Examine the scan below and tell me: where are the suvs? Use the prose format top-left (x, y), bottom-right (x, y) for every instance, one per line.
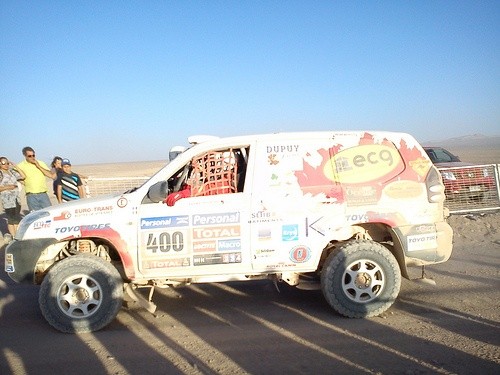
top-left (422, 145), bottom-right (493, 205)
top-left (3, 129), bottom-right (455, 334)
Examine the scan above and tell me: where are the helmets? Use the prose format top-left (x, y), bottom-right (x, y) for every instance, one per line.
top-left (205, 152), bottom-right (235, 178)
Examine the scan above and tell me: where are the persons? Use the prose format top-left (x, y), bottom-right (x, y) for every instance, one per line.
top-left (163, 151), bottom-right (235, 206)
top-left (0, 157), bottom-right (26, 245)
top-left (16, 147), bottom-right (53, 212)
top-left (51, 156), bottom-right (84, 203)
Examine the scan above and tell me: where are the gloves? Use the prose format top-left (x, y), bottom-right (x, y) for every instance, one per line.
top-left (166, 192), bottom-right (185, 206)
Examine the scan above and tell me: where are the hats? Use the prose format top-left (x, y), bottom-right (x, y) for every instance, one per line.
top-left (61, 159), bottom-right (71, 166)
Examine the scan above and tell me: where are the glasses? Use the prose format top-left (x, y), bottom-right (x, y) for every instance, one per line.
top-left (27, 155), bottom-right (35, 158)
top-left (1, 162), bottom-right (9, 165)
